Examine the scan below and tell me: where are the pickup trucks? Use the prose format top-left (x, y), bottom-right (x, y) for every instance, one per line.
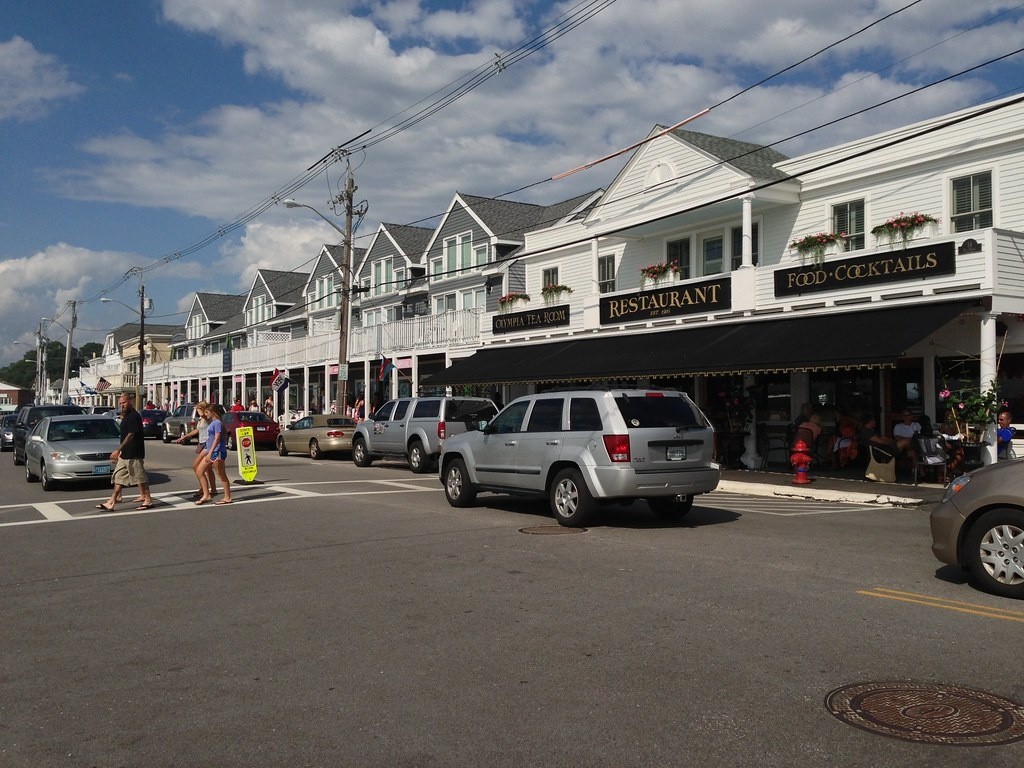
top-left (351, 396), bottom-right (501, 475)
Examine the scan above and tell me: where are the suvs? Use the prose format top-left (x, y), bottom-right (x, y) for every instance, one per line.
top-left (437, 384), bottom-right (721, 528)
top-left (12, 404), bottom-right (89, 467)
top-left (161, 402), bottom-right (200, 445)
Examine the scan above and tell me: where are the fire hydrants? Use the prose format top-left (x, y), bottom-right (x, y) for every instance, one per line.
top-left (788, 438), bottom-right (813, 484)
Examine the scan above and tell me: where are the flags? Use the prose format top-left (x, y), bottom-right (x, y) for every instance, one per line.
top-left (378, 356), bottom-right (395, 381)
top-left (95, 377), bottom-right (111, 391)
top-left (81, 382), bottom-right (97, 395)
top-left (77, 390), bottom-right (84, 402)
top-left (270, 369), bottom-right (290, 393)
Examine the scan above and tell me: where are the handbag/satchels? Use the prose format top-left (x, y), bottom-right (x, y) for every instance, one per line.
top-left (195, 443), bottom-right (205, 454)
top-left (865, 458), bottom-right (896, 483)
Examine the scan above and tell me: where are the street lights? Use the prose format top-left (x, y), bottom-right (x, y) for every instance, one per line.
top-left (99, 297), bottom-right (145, 411)
top-left (13, 340), bottom-right (42, 405)
top-left (282, 197), bottom-right (352, 414)
top-left (41, 317), bottom-right (73, 406)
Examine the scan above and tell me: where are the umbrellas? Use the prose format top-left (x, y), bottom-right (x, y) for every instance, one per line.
top-left (169, 346), bottom-right (177, 361)
top-left (226, 335), bottom-right (233, 350)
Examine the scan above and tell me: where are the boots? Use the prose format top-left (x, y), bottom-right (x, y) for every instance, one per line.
top-left (214, 500), bottom-right (233, 505)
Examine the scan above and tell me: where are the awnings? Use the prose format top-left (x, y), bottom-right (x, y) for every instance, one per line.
top-left (420, 299), bottom-right (980, 389)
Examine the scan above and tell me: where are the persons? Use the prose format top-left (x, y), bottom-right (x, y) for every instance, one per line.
top-left (231, 394), bottom-right (273, 418)
top-left (176, 400), bottom-right (233, 504)
top-left (331, 399), bottom-right (375, 421)
top-left (794, 403), bottom-right (1017, 486)
top-left (95, 392), bottom-right (154, 510)
top-left (146, 394), bottom-right (185, 413)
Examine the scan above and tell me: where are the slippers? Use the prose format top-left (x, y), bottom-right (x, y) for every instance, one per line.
top-left (136, 505), bottom-right (152, 510)
top-left (195, 498), bottom-right (212, 504)
top-left (94, 504), bottom-right (113, 511)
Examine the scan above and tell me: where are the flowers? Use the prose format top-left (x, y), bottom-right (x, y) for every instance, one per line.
top-left (540, 283), bottom-right (575, 308)
top-left (637, 258), bottom-right (683, 295)
top-left (787, 232), bottom-right (846, 272)
top-left (715, 384), bottom-right (753, 432)
top-left (870, 211), bottom-right (942, 253)
top-left (935, 354), bottom-right (1009, 443)
top-left (497, 292), bottom-right (530, 314)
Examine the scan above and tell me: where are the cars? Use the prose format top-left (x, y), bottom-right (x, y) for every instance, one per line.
top-left (275, 414), bottom-right (358, 460)
top-left (81, 406), bottom-right (117, 414)
top-left (138, 409), bottom-right (172, 440)
top-left (929, 454), bottom-right (1024, 599)
top-left (0, 415), bottom-right (17, 452)
top-left (222, 411), bottom-right (280, 451)
top-left (24, 414), bottom-right (124, 491)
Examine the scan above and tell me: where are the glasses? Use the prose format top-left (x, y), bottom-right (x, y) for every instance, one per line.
top-left (998, 419), bottom-right (1004, 421)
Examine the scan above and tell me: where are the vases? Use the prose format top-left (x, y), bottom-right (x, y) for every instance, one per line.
top-left (876, 222), bottom-right (938, 248)
top-left (719, 432), bottom-right (751, 469)
top-left (640, 268), bottom-right (680, 287)
top-left (790, 240), bottom-right (844, 261)
top-left (542, 290), bottom-right (570, 304)
top-left (499, 299), bottom-right (528, 314)
top-left (953, 443), bottom-right (985, 472)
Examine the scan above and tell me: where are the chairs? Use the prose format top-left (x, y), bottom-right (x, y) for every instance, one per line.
top-left (757, 422), bottom-right (789, 470)
top-left (786, 428), bottom-right (818, 474)
top-left (911, 434), bottom-right (949, 486)
